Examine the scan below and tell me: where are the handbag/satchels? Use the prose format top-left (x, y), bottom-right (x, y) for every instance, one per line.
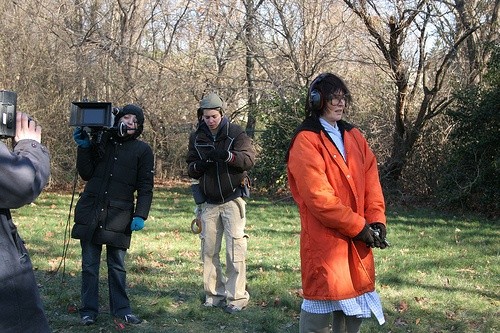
top-left (240, 170), bottom-right (252, 198)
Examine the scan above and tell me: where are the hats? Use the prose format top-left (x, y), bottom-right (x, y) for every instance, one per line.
top-left (199, 93), bottom-right (222, 109)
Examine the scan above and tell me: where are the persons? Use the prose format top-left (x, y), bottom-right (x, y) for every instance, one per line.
top-left (186, 92), bottom-right (255, 314)
top-left (71, 104), bottom-right (154, 325)
top-left (285, 73), bottom-right (387, 333)
top-left (0, 110), bottom-right (54, 333)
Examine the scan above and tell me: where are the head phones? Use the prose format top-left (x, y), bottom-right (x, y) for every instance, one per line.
top-left (196, 96), bottom-right (225, 116)
top-left (307, 72), bottom-right (337, 110)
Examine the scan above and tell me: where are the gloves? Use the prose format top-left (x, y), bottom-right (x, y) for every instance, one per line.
top-left (353, 222), bottom-right (388, 249)
top-left (195, 160), bottom-right (208, 171)
top-left (207, 148), bottom-right (230, 162)
top-left (74, 127), bottom-right (90, 148)
top-left (131, 217), bottom-right (144, 231)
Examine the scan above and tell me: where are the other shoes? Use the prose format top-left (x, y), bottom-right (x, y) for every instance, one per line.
top-left (225, 303), bottom-right (240, 313)
top-left (121, 313), bottom-right (141, 324)
top-left (81, 315), bottom-right (95, 325)
top-left (202, 301), bottom-right (214, 308)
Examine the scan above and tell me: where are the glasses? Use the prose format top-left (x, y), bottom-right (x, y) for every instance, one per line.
top-left (327, 95), bottom-right (347, 106)
